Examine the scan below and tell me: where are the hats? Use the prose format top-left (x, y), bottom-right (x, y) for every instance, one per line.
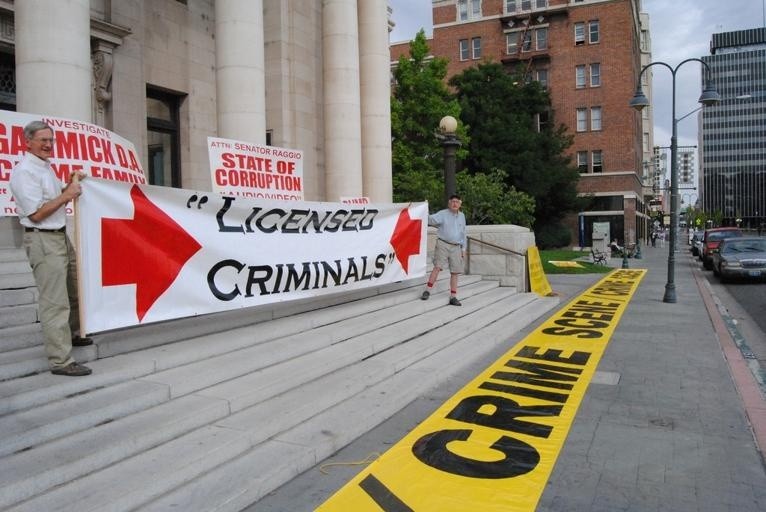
top-left (450, 193), bottom-right (460, 200)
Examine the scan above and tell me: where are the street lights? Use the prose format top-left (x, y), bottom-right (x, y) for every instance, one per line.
top-left (680, 192), bottom-right (701, 220)
top-left (629, 58), bottom-right (722, 305)
top-left (676, 95), bottom-right (752, 132)
top-left (434, 115), bottom-right (461, 211)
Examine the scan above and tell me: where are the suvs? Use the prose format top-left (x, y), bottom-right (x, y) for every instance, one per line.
top-left (700, 227), bottom-right (744, 268)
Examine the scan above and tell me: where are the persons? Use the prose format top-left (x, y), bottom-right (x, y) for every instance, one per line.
top-left (650, 229), bottom-right (657, 248)
top-left (658, 230), bottom-right (666, 248)
top-left (7, 119), bottom-right (95, 377)
top-left (610, 237), bottom-right (625, 254)
top-left (419, 192), bottom-right (469, 307)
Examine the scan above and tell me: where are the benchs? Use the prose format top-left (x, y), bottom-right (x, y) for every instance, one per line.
top-left (591, 248), bottom-right (607, 266)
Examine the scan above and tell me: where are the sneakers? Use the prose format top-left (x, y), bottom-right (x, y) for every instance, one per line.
top-left (420, 291), bottom-right (430, 299)
top-left (71, 336), bottom-right (93, 346)
top-left (52, 361), bottom-right (91, 375)
top-left (450, 297), bottom-right (461, 306)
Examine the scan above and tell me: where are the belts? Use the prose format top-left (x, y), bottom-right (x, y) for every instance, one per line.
top-left (439, 237), bottom-right (459, 246)
top-left (24, 226), bottom-right (66, 233)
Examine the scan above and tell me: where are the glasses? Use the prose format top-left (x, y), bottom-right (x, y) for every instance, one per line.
top-left (33, 137), bottom-right (56, 143)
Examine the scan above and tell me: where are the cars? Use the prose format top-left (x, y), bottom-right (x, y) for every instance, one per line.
top-left (714, 236), bottom-right (766, 284)
top-left (690, 231), bottom-right (704, 261)
